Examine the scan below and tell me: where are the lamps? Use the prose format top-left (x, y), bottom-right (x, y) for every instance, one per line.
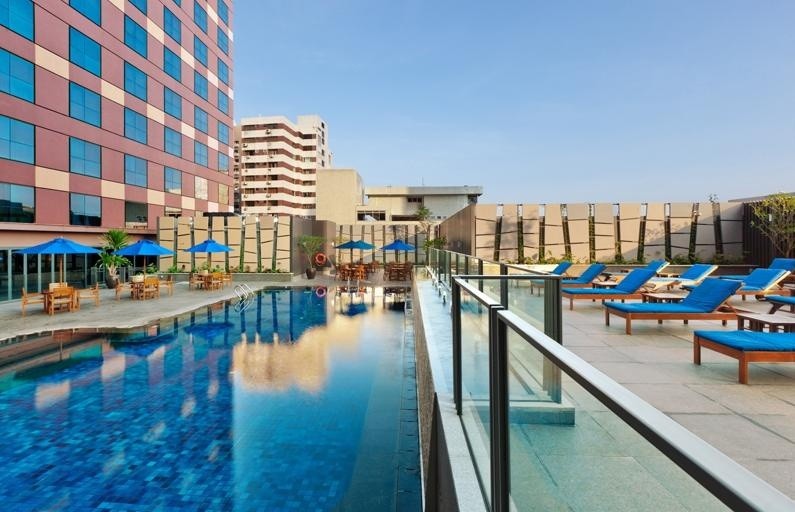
top-left (115, 270), bottom-right (173, 302)
top-left (21, 282), bottom-right (100, 319)
top-left (509, 258), bottom-right (795, 385)
top-left (333, 260), bottom-right (413, 281)
top-left (189, 270), bottom-right (232, 292)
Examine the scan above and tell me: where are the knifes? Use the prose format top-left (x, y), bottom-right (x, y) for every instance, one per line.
top-left (243, 143), bottom-right (248, 147)
top-left (265, 129), bottom-right (270, 133)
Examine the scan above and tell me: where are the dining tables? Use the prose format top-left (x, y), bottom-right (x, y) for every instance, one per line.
top-left (94, 228), bottom-right (134, 288)
top-left (296, 232), bottom-right (329, 279)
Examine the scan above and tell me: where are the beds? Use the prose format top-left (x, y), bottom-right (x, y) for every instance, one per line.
top-left (355, 240), bottom-right (376, 264)
top-left (110, 239), bottom-right (178, 301)
top-left (333, 240), bottom-right (361, 280)
top-left (12, 335), bottom-right (103, 385)
top-left (112, 334), bottom-right (177, 357)
top-left (184, 321), bottom-right (234, 339)
top-left (11, 237), bottom-right (105, 310)
top-left (182, 240), bottom-right (235, 273)
top-left (337, 293), bottom-right (368, 318)
top-left (387, 301), bottom-right (411, 313)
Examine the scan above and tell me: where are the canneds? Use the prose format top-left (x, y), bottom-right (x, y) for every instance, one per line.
top-left (315, 287), bottom-right (327, 298)
top-left (315, 253), bottom-right (326, 264)
